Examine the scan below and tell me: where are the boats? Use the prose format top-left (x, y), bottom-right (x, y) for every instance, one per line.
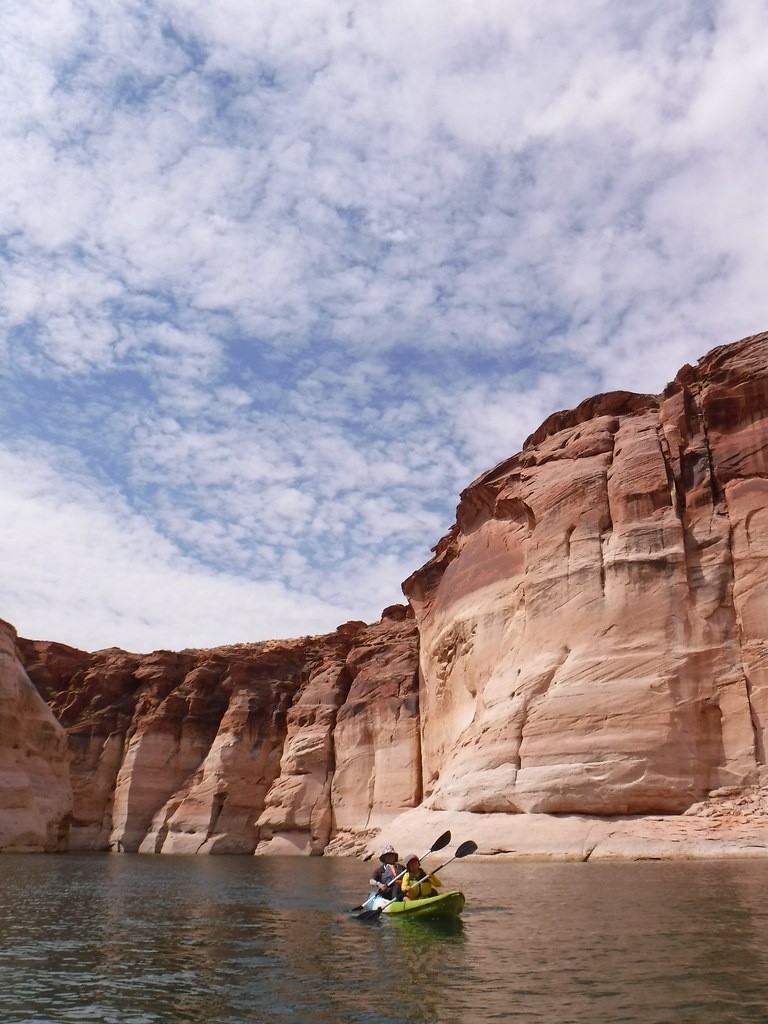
top-left (364, 889), bottom-right (467, 920)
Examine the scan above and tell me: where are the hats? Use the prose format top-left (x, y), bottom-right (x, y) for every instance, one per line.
top-left (379, 844), bottom-right (398, 863)
top-left (405, 854), bottom-right (418, 866)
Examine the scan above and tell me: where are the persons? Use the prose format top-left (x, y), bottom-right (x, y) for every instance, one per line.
top-left (368, 845), bottom-right (441, 902)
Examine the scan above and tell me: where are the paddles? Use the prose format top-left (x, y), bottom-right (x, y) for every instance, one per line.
top-left (351, 830), bottom-right (452, 912)
top-left (357, 840), bottom-right (478, 921)
